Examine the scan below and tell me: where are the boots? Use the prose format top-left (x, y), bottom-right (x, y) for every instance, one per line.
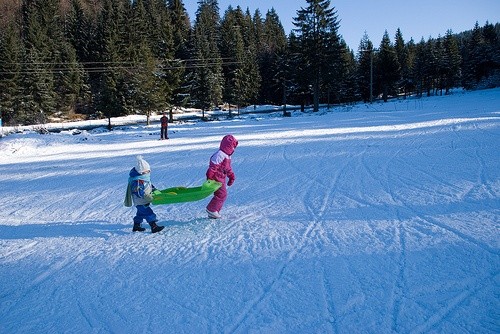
top-left (132, 222), bottom-right (145, 231)
top-left (149, 221), bottom-right (164, 233)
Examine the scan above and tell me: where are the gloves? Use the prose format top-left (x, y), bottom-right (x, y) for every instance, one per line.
top-left (227, 173), bottom-right (235, 186)
top-left (144, 193), bottom-right (154, 202)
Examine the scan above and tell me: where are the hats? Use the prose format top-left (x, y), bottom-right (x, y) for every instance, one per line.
top-left (134, 155), bottom-right (151, 175)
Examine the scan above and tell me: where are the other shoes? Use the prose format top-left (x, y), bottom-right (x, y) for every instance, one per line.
top-left (205, 209), bottom-right (222, 220)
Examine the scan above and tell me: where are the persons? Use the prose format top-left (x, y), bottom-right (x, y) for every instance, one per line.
top-left (206, 134), bottom-right (238, 219)
top-left (128, 155), bottom-right (165, 233)
top-left (160, 113), bottom-right (169, 140)
